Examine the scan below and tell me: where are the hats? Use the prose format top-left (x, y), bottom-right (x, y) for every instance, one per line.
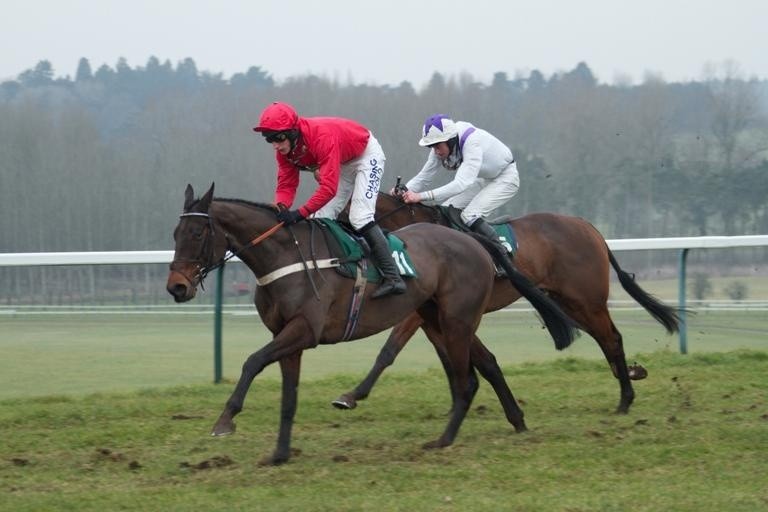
top-left (417, 115), bottom-right (459, 147)
top-left (254, 103), bottom-right (301, 130)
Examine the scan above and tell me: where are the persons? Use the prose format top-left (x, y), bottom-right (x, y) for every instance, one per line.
top-left (253, 103), bottom-right (407, 298)
top-left (391, 115), bottom-right (521, 258)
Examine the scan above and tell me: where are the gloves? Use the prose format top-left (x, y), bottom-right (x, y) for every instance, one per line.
top-left (278, 208), bottom-right (304, 225)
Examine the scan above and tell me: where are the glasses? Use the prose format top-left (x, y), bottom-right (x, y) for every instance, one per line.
top-left (265, 133), bottom-right (287, 143)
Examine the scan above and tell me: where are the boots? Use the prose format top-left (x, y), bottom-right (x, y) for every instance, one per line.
top-left (471, 218), bottom-right (514, 279)
top-left (361, 222), bottom-right (407, 299)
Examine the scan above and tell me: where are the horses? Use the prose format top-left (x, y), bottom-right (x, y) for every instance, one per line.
top-left (165, 181), bottom-right (580, 467)
top-left (331, 187), bottom-right (696, 418)
